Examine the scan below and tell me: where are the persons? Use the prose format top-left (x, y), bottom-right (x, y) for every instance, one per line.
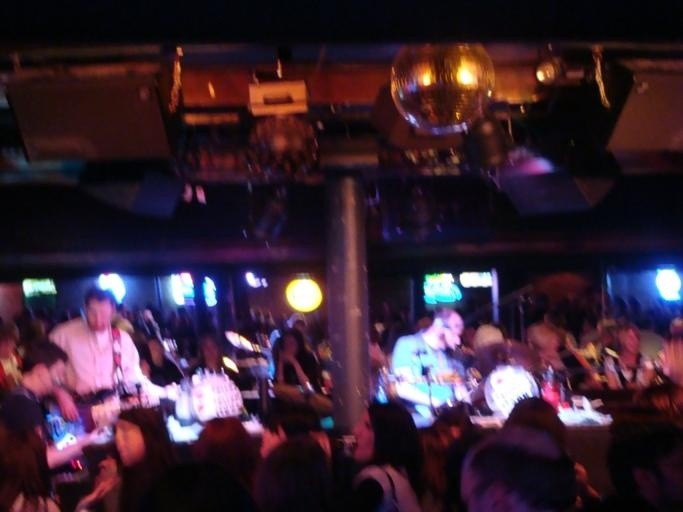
top-left (2, 285), bottom-right (683, 512)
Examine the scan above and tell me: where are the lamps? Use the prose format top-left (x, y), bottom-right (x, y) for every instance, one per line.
top-left (390, 42), bottom-right (495, 135)
top-left (244, 116), bottom-right (318, 181)
top-left (536, 55), bottom-right (569, 85)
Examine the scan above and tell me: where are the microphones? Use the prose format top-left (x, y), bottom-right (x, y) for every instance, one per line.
top-left (142, 307), bottom-right (161, 335)
